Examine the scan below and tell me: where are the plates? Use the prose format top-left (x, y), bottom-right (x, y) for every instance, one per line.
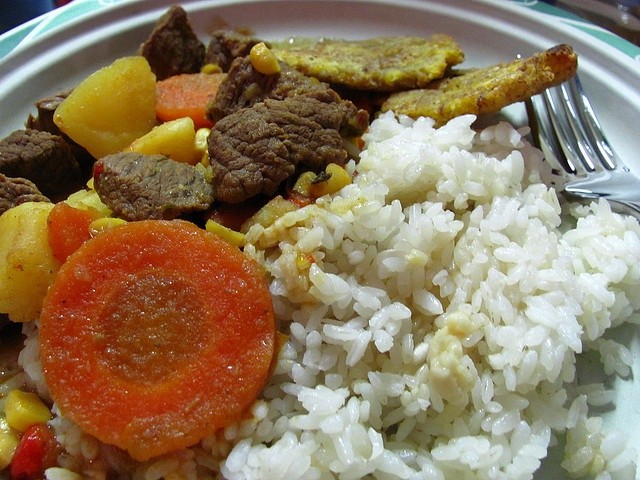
top-left (0, 1), bottom-right (638, 476)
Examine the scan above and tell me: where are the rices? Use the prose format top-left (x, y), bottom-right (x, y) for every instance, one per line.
top-left (19, 109), bottom-right (640, 480)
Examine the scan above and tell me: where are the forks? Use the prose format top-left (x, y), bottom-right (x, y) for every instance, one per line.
top-left (515, 51), bottom-right (640, 223)
